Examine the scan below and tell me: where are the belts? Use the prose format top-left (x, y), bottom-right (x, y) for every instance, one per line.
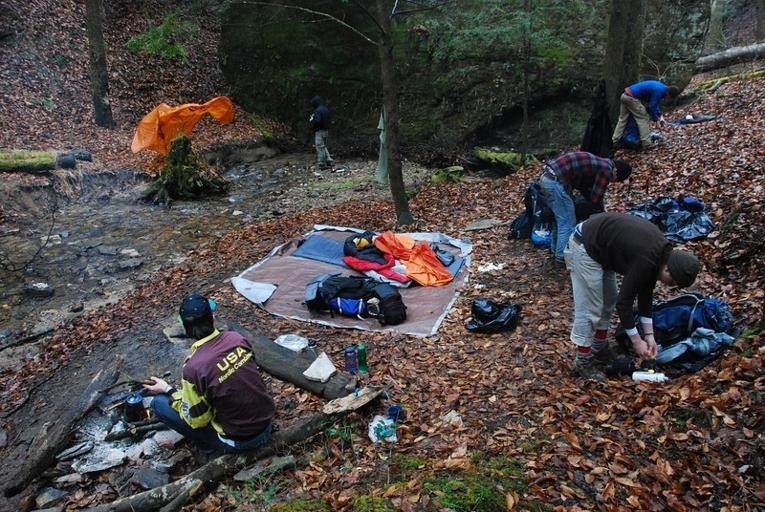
top-left (226, 423), bottom-right (271, 442)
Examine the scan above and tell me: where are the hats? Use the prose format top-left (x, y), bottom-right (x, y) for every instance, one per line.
top-left (614, 160), bottom-right (631, 182)
top-left (669, 86), bottom-right (680, 98)
top-left (178, 294), bottom-right (216, 317)
top-left (667, 250), bottom-right (700, 288)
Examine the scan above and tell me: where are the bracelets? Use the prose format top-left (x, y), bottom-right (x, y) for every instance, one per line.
top-left (643, 332), bottom-right (655, 336)
top-left (167, 388), bottom-right (178, 396)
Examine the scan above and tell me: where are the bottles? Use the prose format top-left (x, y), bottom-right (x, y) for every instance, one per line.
top-left (632, 372), bottom-right (667, 384)
top-left (125, 394), bottom-right (147, 422)
top-left (345, 345), bottom-right (366, 376)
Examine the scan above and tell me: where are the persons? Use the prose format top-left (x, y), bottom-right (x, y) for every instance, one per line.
top-left (611, 79), bottom-right (680, 150)
top-left (306, 95), bottom-right (334, 166)
top-left (540, 151), bottom-right (633, 265)
top-left (141, 292), bottom-right (277, 456)
top-left (561, 209), bottom-right (701, 382)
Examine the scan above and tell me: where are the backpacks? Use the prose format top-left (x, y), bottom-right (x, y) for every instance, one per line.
top-left (616, 292), bottom-right (731, 348)
top-left (303, 272), bottom-right (406, 326)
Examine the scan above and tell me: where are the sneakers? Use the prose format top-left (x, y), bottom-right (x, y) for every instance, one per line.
top-left (551, 254), bottom-right (565, 264)
top-left (572, 339), bottom-right (620, 382)
